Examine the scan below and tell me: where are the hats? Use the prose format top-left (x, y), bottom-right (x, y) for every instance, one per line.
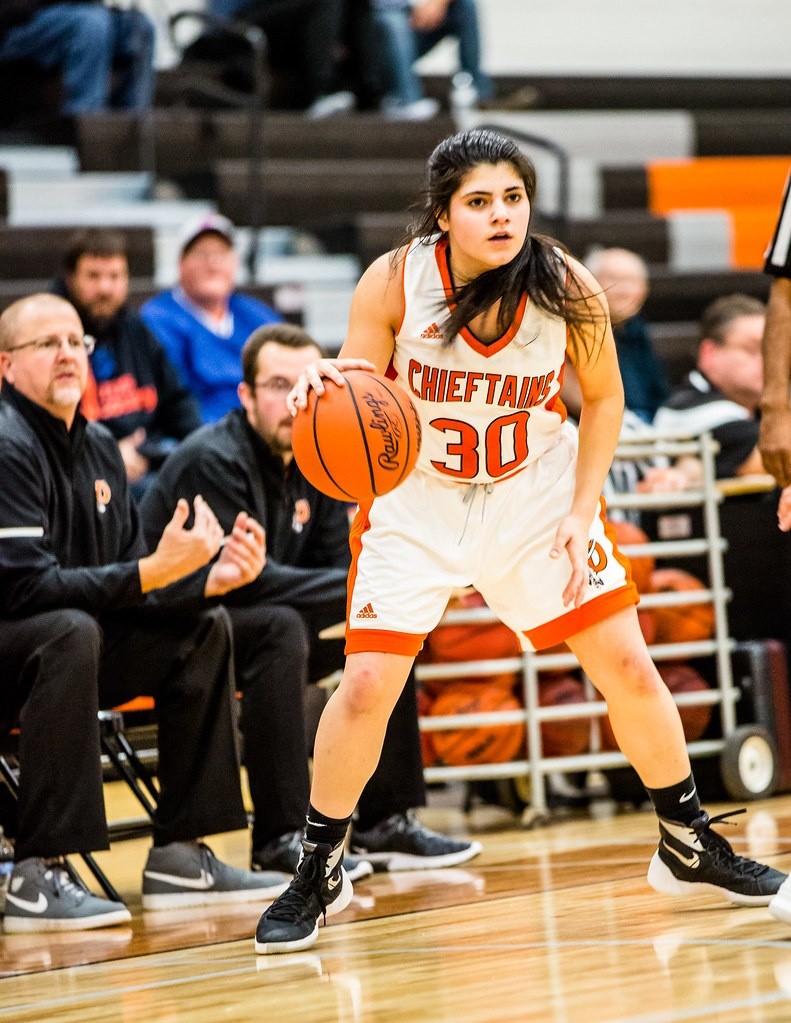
top-left (179, 214), bottom-right (233, 257)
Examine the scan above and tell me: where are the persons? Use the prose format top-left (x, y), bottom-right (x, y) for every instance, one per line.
top-left (0, 213), bottom-right (487, 938)
top-left (0, 0), bottom-right (539, 118)
top-left (252, 129), bottom-right (787, 957)
top-left (559, 168), bottom-right (791, 926)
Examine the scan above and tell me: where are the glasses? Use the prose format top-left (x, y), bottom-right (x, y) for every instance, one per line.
top-left (6, 334), bottom-right (96, 356)
top-left (725, 339), bottom-right (761, 356)
top-left (255, 378), bottom-right (293, 394)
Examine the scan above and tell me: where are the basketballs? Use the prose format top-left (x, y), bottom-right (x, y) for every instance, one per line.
top-left (416, 688), bottom-right (436, 767)
top-left (637, 608), bottom-right (658, 643)
top-left (649, 568), bottom-right (714, 639)
top-left (420, 646), bottom-right (517, 695)
top-left (428, 585), bottom-right (517, 663)
top-left (426, 683), bottom-right (525, 766)
top-left (291, 369), bottom-right (422, 503)
top-left (658, 664), bottom-right (714, 742)
top-left (540, 677), bottom-right (592, 754)
top-left (538, 642), bottom-right (574, 678)
top-left (595, 689), bottom-right (620, 750)
top-left (609, 521), bottom-right (653, 593)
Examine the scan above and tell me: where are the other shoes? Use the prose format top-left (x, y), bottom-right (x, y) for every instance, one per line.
top-left (305, 90), bottom-right (354, 120)
top-left (383, 95), bottom-right (438, 122)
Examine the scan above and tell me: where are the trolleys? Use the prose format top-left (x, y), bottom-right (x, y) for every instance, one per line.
top-left (319, 426), bottom-right (777, 827)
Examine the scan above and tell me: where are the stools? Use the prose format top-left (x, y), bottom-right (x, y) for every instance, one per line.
top-left (1, 709), bottom-right (163, 907)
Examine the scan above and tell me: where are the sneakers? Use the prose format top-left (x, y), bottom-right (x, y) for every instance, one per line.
top-left (647, 808), bottom-right (788, 906)
top-left (344, 807), bottom-right (483, 869)
top-left (768, 872), bottom-right (791, 925)
top-left (255, 834), bottom-right (353, 953)
top-left (249, 828), bottom-right (373, 884)
top-left (4, 858), bottom-right (133, 933)
top-left (141, 844), bottom-right (292, 909)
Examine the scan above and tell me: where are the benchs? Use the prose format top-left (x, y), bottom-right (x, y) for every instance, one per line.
top-left (0, 67), bottom-right (790, 390)
